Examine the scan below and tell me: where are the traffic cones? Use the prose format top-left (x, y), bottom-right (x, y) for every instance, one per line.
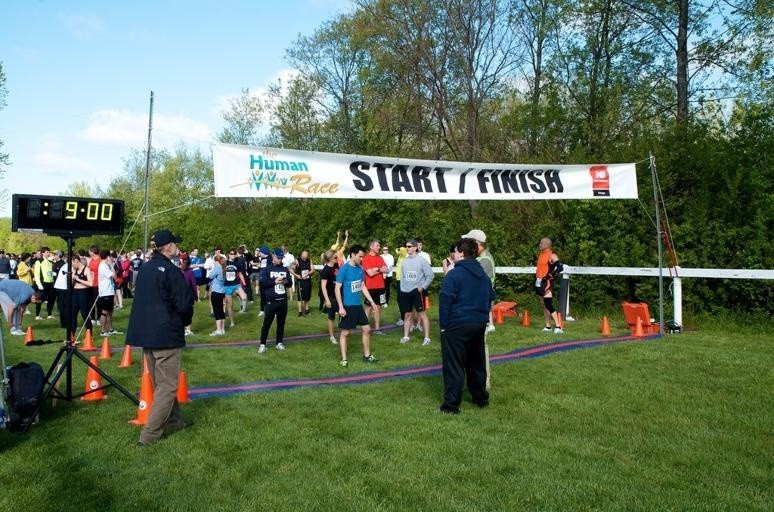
top-left (492, 308), bottom-right (506, 324)
top-left (78, 328), bottom-right (96, 351)
top-left (23, 326), bottom-right (34, 345)
top-left (127, 371), bottom-right (154, 425)
top-left (65, 331), bottom-right (75, 348)
top-left (175, 370), bottom-right (192, 405)
top-left (138, 353), bottom-right (150, 381)
top-left (630, 315), bottom-right (648, 338)
top-left (424, 296), bottom-right (431, 310)
top-left (519, 309), bottom-right (532, 327)
top-left (95, 336), bottom-right (114, 360)
top-left (116, 344), bottom-right (132, 368)
top-left (553, 312), bottom-right (565, 330)
top-left (79, 355), bottom-right (107, 401)
top-left (599, 315), bottom-right (612, 337)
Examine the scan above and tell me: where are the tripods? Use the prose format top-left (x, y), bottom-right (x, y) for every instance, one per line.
top-left (22, 247), bottom-right (140, 433)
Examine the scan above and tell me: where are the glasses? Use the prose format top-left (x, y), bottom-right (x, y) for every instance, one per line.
top-left (405, 246), bottom-right (415, 248)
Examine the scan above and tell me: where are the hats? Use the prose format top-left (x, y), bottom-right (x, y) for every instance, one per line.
top-left (52, 250), bottom-right (63, 257)
top-left (153, 229), bottom-right (183, 247)
top-left (272, 247), bottom-right (285, 258)
top-left (460, 229), bottom-right (486, 243)
top-left (259, 246), bottom-right (270, 255)
top-left (406, 239), bottom-right (419, 252)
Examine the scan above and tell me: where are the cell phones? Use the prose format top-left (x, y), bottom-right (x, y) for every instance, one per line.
top-left (281, 277), bottom-right (286, 281)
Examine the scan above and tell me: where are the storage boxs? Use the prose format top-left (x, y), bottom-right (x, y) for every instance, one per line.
top-left (621, 299), bottom-right (662, 337)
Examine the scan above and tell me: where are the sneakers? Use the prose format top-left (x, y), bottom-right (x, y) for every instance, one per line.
top-left (542, 327), bottom-right (552, 331)
top-left (399, 336), bottom-right (410, 343)
top-left (91, 319), bottom-right (123, 337)
top-left (487, 324), bottom-right (495, 331)
top-left (257, 343), bottom-right (265, 353)
top-left (10, 311), bottom-right (55, 336)
top-left (298, 312), bottom-right (306, 318)
top-left (275, 343), bottom-right (286, 350)
top-left (184, 321), bottom-right (236, 336)
top-left (396, 319), bottom-right (424, 332)
top-left (422, 337), bottom-right (432, 346)
top-left (554, 327), bottom-right (563, 334)
top-left (304, 309), bottom-right (311, 316)
top-left (341, 359), bottom-right (348, 367)
top-left (363, 354), bottom-right (380, 363)
top-left (329, 336), bottom-right (338, 344)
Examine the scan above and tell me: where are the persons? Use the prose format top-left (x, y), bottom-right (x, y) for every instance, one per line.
top-left (438, 238), bottom-right (488, 414)
top-left (319, 230), bottom-right (394, 344)
top-left (443, 244), bottom-right (457, 274)
top-left (287, 251), bottom-right (314, 317)
top-left (172, 244), bottom-right (273, 336)
top-left (334, 245), bottom-right (380, 366)
top-left (395, 240), bottom-right (434, 346)
top-left (258, 247), bottom-right (292, 354)
top-left (0, 245), bottom-right (150, 336)
top-left (534, 238), bottom-right (563, 334)
top-left (461, 229), bottom-right (495, 392)
top-left (125, 230), bottom-right (193, 446)
